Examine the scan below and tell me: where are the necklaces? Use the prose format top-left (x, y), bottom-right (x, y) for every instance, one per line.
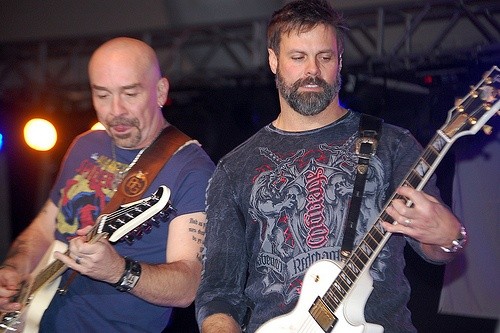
top-left (110, 141), bottom-right (145, 190)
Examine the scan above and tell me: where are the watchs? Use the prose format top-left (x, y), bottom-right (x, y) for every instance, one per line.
top-left (441, 226), bottom-right (468, 255)
top-left (114, 257), bottom-right (141, 292)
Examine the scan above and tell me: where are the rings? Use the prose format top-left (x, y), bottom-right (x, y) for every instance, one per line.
top-left (76, 257), bottom-right (80, 264)
top-left (404, 220), bottom-right (411, 227)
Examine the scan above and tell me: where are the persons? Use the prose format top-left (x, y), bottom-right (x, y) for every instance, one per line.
top-left (195, 0), bottom-right (463, 333)
top-left (0, 37), bottom-right (215, 333)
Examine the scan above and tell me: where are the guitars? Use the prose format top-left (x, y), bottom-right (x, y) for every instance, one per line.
top-left (0, 185), bottom-right (178, 333)
top-left (253, 63), bottom-right (500, 333)
